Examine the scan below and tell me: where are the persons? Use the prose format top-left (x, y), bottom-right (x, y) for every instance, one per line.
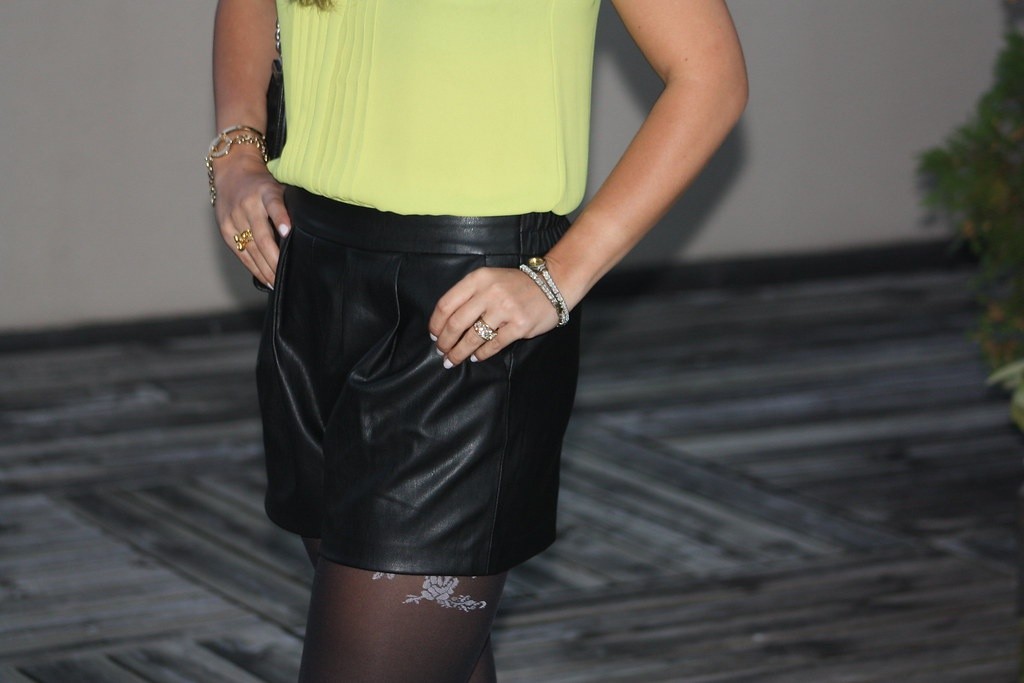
top-left (205, 0), bottom-right (749, 683)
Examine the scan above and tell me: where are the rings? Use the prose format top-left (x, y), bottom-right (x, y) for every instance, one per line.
top-left (234, 229), bottom-right (255, 252)
top-left (473, 315), bottom-right (498, 341)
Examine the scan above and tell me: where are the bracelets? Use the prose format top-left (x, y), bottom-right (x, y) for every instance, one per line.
top-left (519, 257), bottom-right (570, 327)
top-left (206, 125), bottom-right (268, 208)
